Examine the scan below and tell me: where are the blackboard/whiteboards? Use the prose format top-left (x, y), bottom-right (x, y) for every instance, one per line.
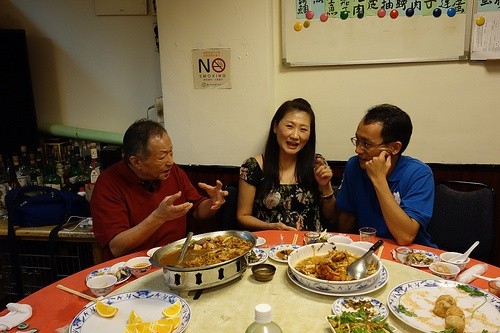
top-left (279, 0), bottom-right (475, 68)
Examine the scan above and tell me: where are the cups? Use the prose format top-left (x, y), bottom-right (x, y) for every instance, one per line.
top-left (395, 246), bottom-right (413, 267)
top-left (304, 232), bottom-right (321, 245)
top-left (487, 279), bottom-right (500, 298)
top-left (359, 227), bottom-right (377, 243)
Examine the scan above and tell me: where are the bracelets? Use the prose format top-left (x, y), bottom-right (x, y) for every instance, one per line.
top-left (320, 190), bottom-right (334, 200)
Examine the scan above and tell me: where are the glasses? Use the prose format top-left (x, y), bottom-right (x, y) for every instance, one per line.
top-left (351, 137), bottom-right (392, 150)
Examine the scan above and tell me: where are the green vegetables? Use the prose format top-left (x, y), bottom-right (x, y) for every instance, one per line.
top-left (431, 328), bottom-right (489, 333)
top-left (398, 305), bottom-right (418, 317)
top-left (456, 283), bottom-right (488, 297)
top-left (327, 309), bottom-right (392, 333)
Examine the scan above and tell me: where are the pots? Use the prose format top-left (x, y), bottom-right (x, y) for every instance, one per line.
top-left (151, 230), bottom-right (257, 290)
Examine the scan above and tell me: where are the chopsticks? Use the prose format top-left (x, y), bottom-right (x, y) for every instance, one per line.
top-left (55, 284), bottom-right (96, 301)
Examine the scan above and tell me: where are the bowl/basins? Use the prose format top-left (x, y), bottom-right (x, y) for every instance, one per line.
top-left (126, 247), bottom-right (162, 277)
top-left (440, 252), bottom-right (470, 271)
top-left (327, 236), bottom-right (374, 251)
top-left (325, 315), bottom-right (397, 333)
top-left (86, 275), bottom-right (117, 297)
top-left (252, 263), bottom-right (276, 281)
top-left (287, 242), bottom-right (382, 292)
top-left (429, 262), bottom-right (461, 281)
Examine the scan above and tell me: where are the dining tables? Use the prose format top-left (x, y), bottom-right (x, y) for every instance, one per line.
top-left (0, 229), bottom-right (500, 333)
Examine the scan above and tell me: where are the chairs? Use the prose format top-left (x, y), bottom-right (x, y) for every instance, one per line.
top-left (427, 180), bottom-right (494, 265)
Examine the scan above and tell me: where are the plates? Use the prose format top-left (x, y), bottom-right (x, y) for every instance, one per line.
top-left (286, 266), bottom-right (389, 297)
top-left (331, 296), bottom-right (389, 322)
top-left (247, 237), bottom-right (303, 264)
top-left (69, 289), bottom-right (191, 333)
top-left (86, 266), bottom-right (131, 285)
top-left (392, 249), bottom-right (439, 268)
top-left (387, 279), bottom-right (500, 333)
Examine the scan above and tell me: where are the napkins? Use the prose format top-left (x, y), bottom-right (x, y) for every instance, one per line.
top-left (0, 303), bottom-right (32, 331)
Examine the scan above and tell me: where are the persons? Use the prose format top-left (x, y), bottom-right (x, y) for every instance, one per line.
top-left (90, 118), bottom-right (229, 261)
top-left (237, 97), bottom-right (337, 231)
top-left (334, 103), bottom-right (438, 248)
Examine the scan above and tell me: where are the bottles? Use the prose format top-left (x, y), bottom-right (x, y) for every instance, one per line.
top-left (245, 304), bottom-right (282, 333)
top-left (0, 140), bottom-right (102, 221)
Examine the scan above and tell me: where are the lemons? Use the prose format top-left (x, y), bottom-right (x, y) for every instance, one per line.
top-left (96, 301), bottom-right (119, 317)
top-left (125, 300), bottom-right (185, 333)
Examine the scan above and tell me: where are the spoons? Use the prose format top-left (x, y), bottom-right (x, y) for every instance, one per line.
top-left (447, 241), bottom-right (479, 263)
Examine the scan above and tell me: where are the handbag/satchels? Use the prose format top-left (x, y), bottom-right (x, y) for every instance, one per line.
top-left (5, 185), bottom-right (87, 228)
